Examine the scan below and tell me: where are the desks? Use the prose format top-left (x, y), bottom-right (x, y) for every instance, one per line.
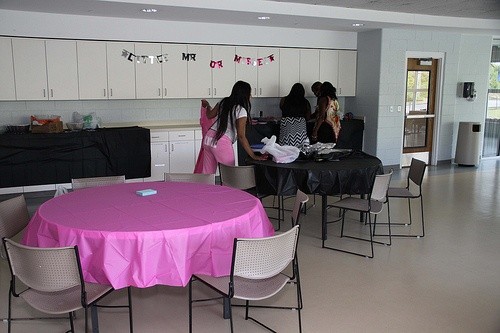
top-left (246, 150), bottom-right (383, 241)
top-left (22, 182), bottom-right (275, 291)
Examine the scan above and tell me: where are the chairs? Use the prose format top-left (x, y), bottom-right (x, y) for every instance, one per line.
top-left (0, 158), bottom-right (428, 333)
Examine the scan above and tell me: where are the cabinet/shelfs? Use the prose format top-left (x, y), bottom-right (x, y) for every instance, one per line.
top-left (97, 120), bottom-right (240, 181)
top-left (0, 34), bottom-right (357, 102)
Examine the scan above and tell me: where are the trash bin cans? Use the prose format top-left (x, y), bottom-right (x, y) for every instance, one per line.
top-left (454, 121), bottom-right (482, 166)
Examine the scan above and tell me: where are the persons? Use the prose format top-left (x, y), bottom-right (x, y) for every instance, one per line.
top-left (309, 82), bottom-right (342, 145)
top-left (279, 83), bottom-right (311, 148)
top-left (201, 81), bottom-right (268, 174)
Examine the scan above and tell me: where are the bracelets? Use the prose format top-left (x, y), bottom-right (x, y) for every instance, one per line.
top-left (205, 105), bottom-right (210, 108)
